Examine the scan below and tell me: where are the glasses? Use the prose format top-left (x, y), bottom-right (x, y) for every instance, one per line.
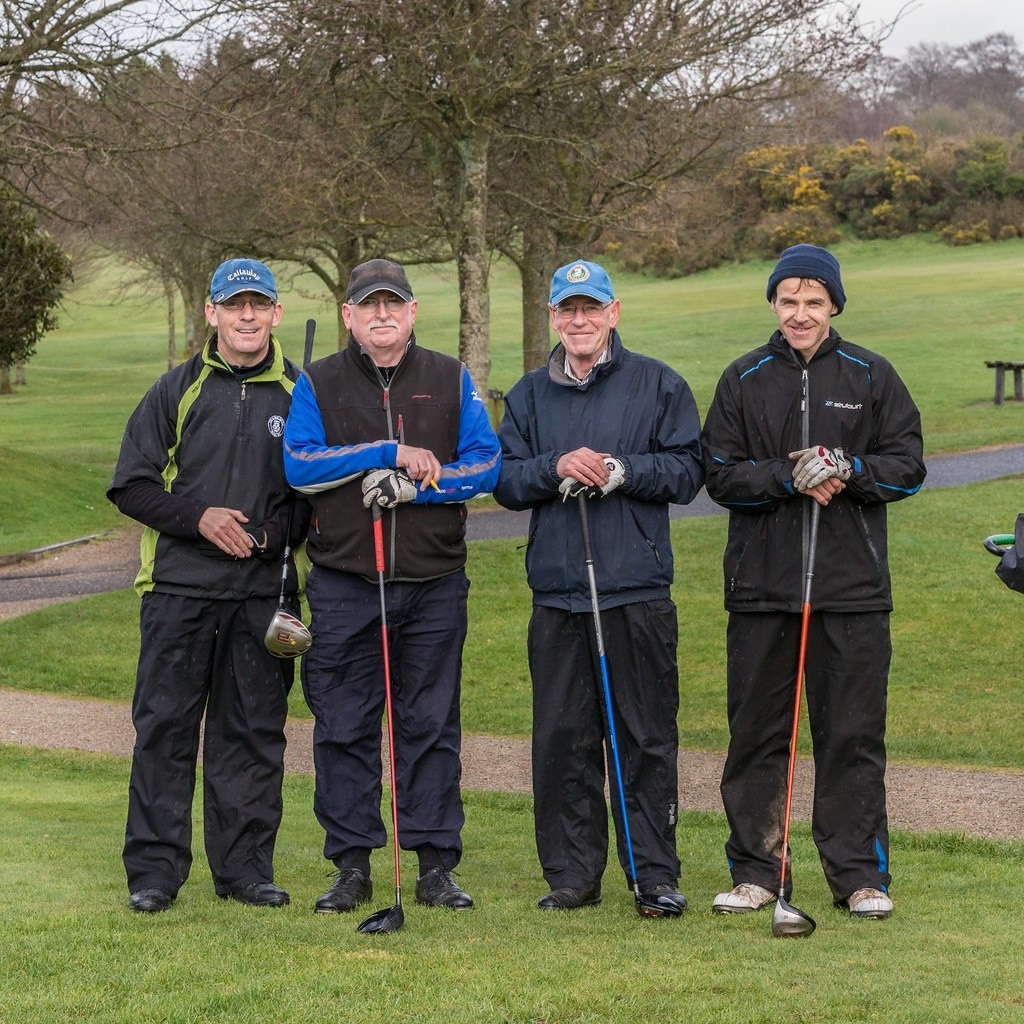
top-left (348, 297), bottom-right (405, 312)
top-left (552, 301), bottom-right (614, 319)
top-left (215, 297), bottom-right (275, 311)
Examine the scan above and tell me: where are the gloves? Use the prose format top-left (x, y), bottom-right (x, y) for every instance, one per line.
top-left (558, 458), bottom-right (626, 504)
top-left (361, 468), bottom-right (417, 509)
top-left (789, 445), bottom-right (852, 491)
top-left (198, 524), bottom-right (268, 562)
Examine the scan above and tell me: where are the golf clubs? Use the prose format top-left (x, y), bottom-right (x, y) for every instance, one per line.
top-left (354, 494), bottom-right (405, 937)
top-left (263, 318), bottom-right (316, 660)
top-left (770, 498), bottom-right (822, 940)
top-left (574, 492), bottom-right (690, 918)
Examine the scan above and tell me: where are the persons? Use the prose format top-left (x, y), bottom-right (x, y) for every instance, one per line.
top-left (283, 259), bottom-right (501, 917)
top-left (699, 243), bottom-right (927, 919)
top-left (494, 261), bottom-right (706, 915)
top-left (105, 258), bottom-right (312, 913)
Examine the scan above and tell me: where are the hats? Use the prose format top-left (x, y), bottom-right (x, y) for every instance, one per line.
top-left (346, 259), bottom-right (414, 305)
top-left (549, 259), bottom-right (615, 307)
top-left (767, 244), bottom-right (847, 317)
top-left (210, 258), bottom-right (277, 304)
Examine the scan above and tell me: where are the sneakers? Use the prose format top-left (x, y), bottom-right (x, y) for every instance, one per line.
top-left (644, 884), bottom-right (687, 909)
top-left (414, 866), bottom-right (473, 907)
top-left (220, 881), bottom-right (290, 906)
top-left (316, 868), bottom-right (374, 914)
top-left (847, 888), bottom-right (893, 919)
top-left (712, 883), bottom-right (777, 913)
top-left (129, 888), bottom-right (174, 911)
top-left (538, 888), bottom-right (602, 909)
